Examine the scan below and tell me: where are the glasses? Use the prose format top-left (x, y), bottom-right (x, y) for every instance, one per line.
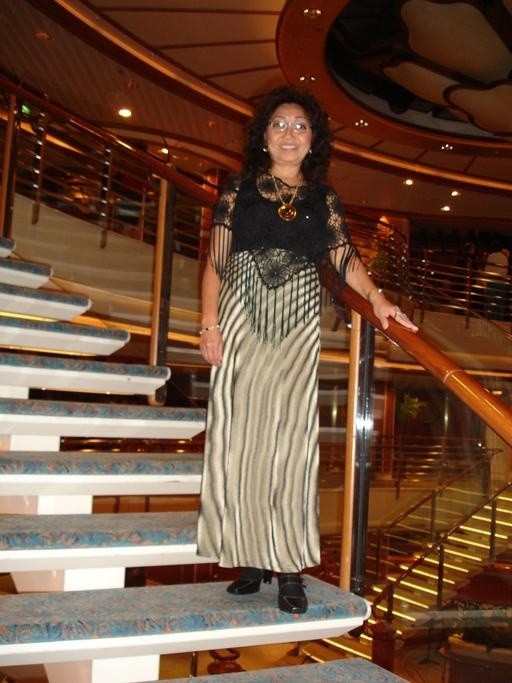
top-left (265, 117), bottom-right (313, 133)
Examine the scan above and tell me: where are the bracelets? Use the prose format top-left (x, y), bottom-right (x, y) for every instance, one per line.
top-left (367, 286), bottom-right (384, 305)
top-left (198, 324), bottom-right (220, 335)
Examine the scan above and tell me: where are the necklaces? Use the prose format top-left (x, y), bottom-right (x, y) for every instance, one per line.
top-left (266, 160), bottom-right (307, 222)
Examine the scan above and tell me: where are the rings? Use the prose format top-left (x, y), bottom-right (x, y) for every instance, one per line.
top-left (393, 310), bottom-right (401, 319)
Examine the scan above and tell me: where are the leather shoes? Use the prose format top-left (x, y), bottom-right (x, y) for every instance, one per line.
top-left (277, 572), bottom-right (308, 614)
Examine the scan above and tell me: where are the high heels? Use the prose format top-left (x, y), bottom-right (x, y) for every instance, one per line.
top-left (226, 564), bottom-right (273, 595)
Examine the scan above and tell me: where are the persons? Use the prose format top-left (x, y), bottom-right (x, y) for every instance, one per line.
top-left (196, 81), bottom-right (421, 616)
top-left (484, 247), bottom-right (510, 319)
top-left (441, 240), bottom-right (482, 317)
